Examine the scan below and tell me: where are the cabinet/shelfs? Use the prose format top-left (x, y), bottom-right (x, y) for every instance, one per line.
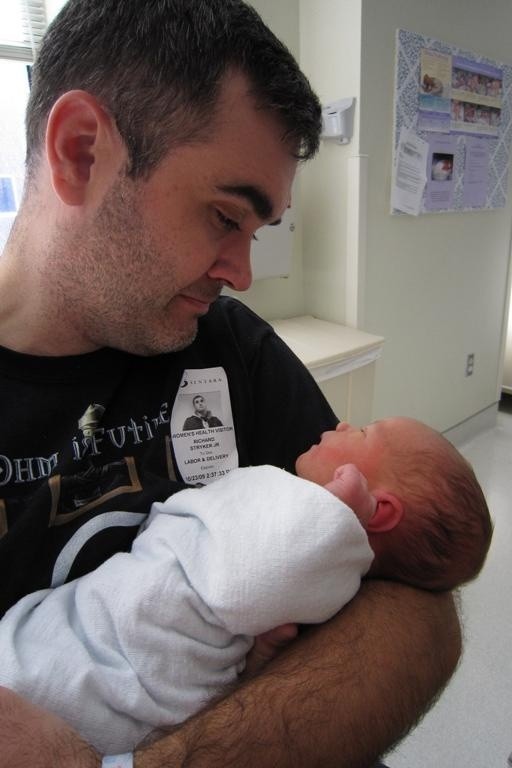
top-left (268, 315), bottom-right (387, 436)
